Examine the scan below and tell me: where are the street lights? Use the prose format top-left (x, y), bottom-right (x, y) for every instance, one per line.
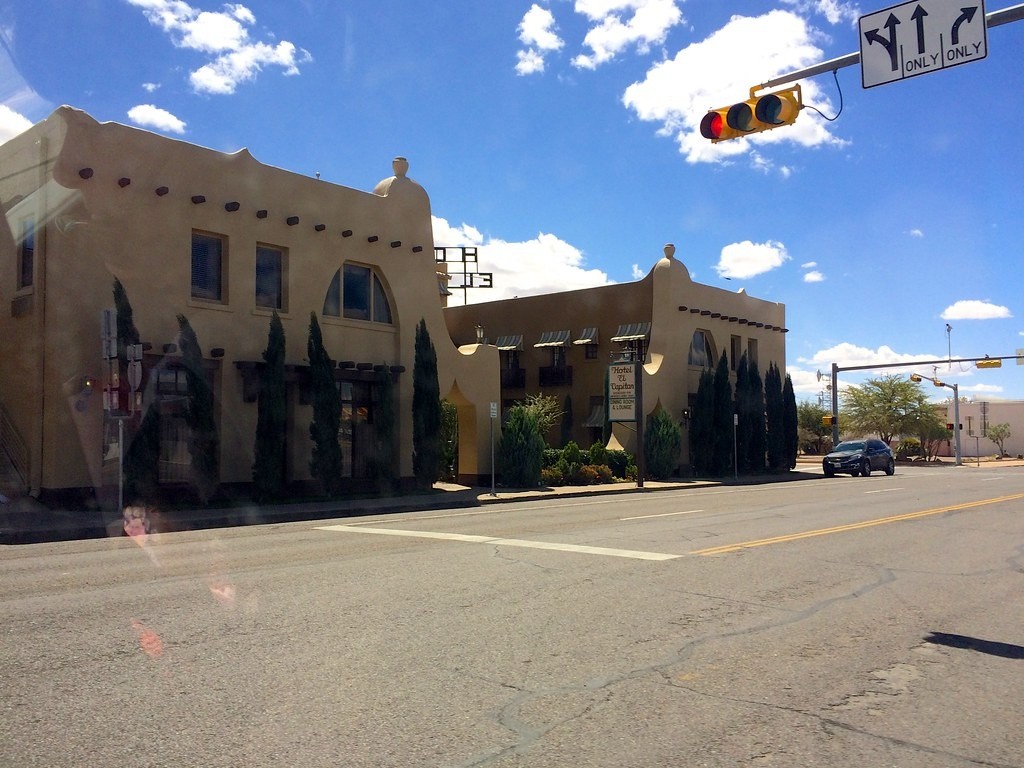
top-left (475, 324), bottom-right (486, 344)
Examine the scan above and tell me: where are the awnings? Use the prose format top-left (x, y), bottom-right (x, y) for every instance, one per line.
top-left (495, 334), bottom-right (525, 351)
top-left (534, 331), bottom-right (573, 348)
top-left (610, 321), bottom-right (652, 344)
top-left (573, 327), bottom-right (600, 345)
top-left (581, 404), bottom-right (606, 428)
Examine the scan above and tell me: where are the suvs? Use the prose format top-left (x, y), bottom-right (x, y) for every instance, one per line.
top-left (823, 438), bottom-right (895, 477)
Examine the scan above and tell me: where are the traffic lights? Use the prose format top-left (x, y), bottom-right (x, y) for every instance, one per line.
top-left (700, 83), bottom-right (804, 143)
top-left (947, 424), bottom-right (954, 430)
